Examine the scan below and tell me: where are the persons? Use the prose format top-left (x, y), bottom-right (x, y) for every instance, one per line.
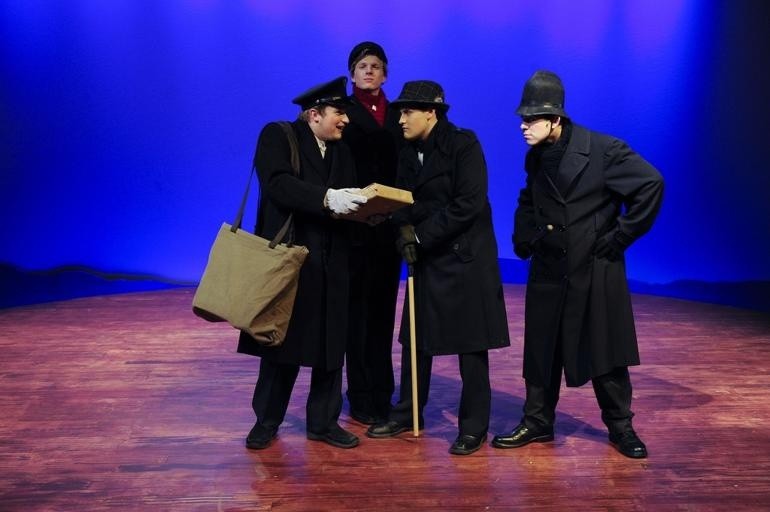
top-left (491, 70), bottom-right (665, 458)
top-left (236, 75), bottom-right (395, 450)
top-left (330, 42), bottom-right (405, 426)
top-left (364, 80), bottom-right (512, 456)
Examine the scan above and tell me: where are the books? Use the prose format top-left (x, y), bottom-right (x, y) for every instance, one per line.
top-left (332, 183), bottom-right (414, 227)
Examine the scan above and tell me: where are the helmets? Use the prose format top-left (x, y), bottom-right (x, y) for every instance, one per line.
top-left (517, 70), bottom-right (571, 118)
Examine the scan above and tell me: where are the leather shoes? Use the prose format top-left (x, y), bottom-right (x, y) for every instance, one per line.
top-left (245, 421), bottom-right (279, 449)
top-left (448, 431), bottom-right (487, 455)
top-left (306, 423), bottom-right (359, 449)
top-left (367, 410), bottom-right (425, 438)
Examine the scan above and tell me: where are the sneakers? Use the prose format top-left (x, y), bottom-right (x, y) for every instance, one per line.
top-left (491, 423), bottom-right (554, 449)
top-left (608, 426), bottom-right (648, 458)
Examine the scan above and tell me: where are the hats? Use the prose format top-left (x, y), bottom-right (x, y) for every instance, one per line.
top-left (388, 80), bottom-right (450, 111)
top-left (348, 41), bottom-right (387, 72)
top-left (291, 75), bottom-right (356, 112)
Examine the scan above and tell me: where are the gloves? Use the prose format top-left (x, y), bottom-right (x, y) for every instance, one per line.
top-left (395, 224), bottom-right (416, 251)
top-left (324, 187), bottom-right (367, 216)
top-left (592, 226), bottom-right (634, 259)
top-left (402, 244), bottom-right (418, 265)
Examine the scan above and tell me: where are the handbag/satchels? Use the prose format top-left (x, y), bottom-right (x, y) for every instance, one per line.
top-left (191, 221), bottom-right (309, 348)
